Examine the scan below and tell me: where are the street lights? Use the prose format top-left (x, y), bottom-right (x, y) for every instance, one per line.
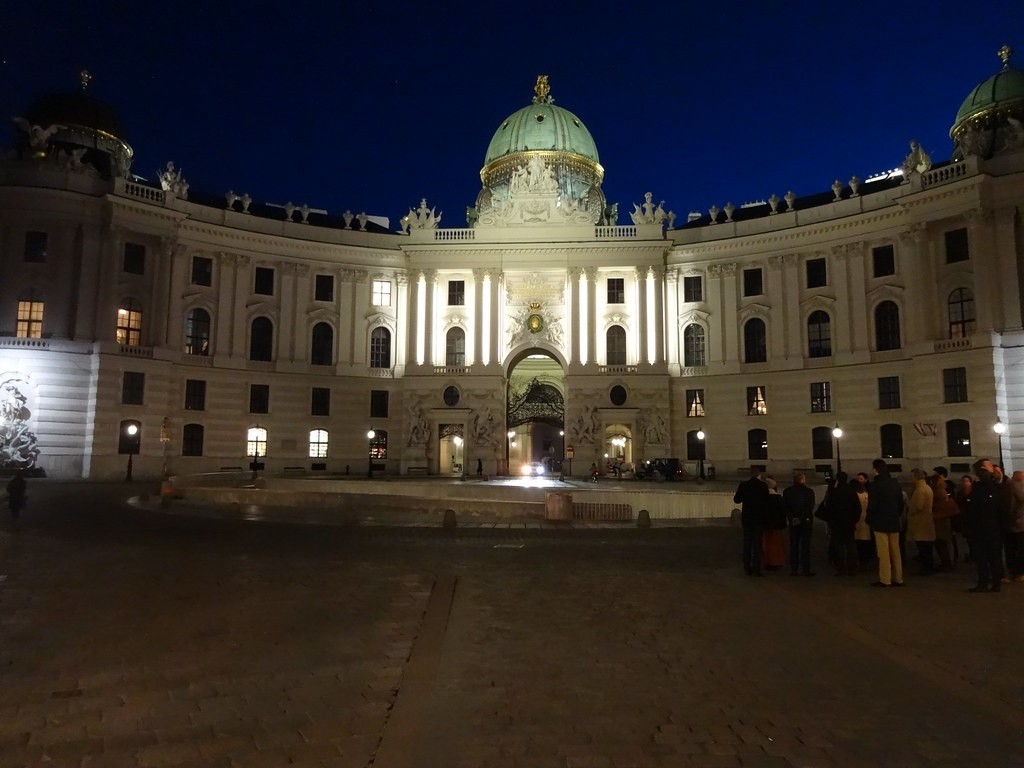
top-left (126, 424), bottom-right (138, 483)
top-left (995, 416), bottom-right (1006, 477)
top-left (696, 427), bottom-right (707, 482)
top-left (831, 422), bottom-right (843, 475)
top-left (367, 425), bottom-right (375, 480)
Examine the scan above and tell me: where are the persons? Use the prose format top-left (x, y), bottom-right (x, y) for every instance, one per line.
top-left (511, 153), bottom-right (558, 192)
top-left (906, 140), bottom-right (923, 174)
top-left (587, 458), bottom-right (668, 483)
top-left (732, 460), bottom-right (1024, 593)
top-left (164, 162), bottom-right (189, 201)
top-left (7, 470), bottom-right (26, 517)
top-left (642, 192), bottom-right (655, 223)
top-left (477, 458), bottom-right (482, 478)
top-left (417, 199), bottom-right (431, 225)
top-left (450, 455), bottom-right (455, 472)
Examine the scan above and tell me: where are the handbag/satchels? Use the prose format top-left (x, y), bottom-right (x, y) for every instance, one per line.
top-left (161, 487), bottom-right (173, 497)
top-left (933, 487), bottom-right (960, 519)
top-left (814, 488), bottom-right (841, 522)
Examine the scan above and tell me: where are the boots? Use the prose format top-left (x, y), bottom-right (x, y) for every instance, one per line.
top-left (968, 570), bottom-right (987, 592)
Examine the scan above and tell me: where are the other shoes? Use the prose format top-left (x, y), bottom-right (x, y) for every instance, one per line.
top-left (935, 564), bottom-right (950, 573)
top-left (801, 572), bottom-right (815, 576)
top-left (920, 569), bottom-right (934, 576)
top-left (790, 572), bottom-right (798, 576)
top-left (893, 581), bottom-right (903, 586)
top-left (748, 569), bottom-right (753, 575)
top-left (880, 581), bottom-right (891, 589)
top-left (754, 572), bottom-right (764, 576)
top-left (1002, 572), bottom-right (1024, 583)
